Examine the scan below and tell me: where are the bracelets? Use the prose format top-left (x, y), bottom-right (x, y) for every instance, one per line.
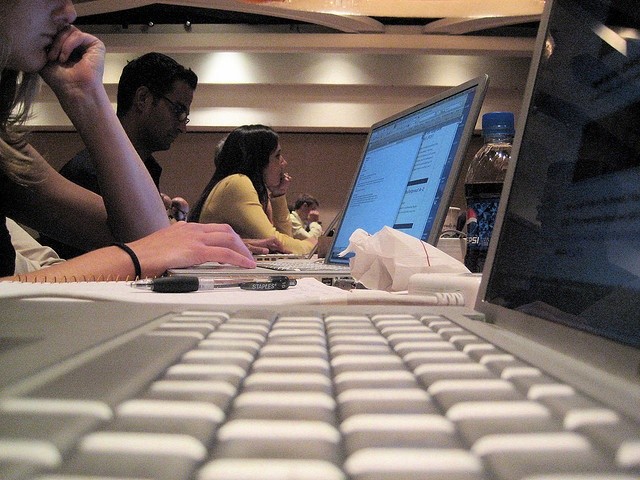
top-left (110, 240), bottom-right (142, 280)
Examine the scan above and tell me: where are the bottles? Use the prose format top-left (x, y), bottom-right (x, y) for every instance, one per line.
top-left (463, 111), bottom-right (515, 273)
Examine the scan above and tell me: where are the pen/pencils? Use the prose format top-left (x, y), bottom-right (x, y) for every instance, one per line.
top-left (131, 276), bottom-right (298, 291)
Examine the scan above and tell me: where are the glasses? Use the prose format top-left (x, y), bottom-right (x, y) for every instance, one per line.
top-left (176, 207), bottom-right (188, 216)
top-left (151, 87), bottom-right (190, 124)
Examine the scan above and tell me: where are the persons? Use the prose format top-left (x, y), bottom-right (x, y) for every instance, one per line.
top-left (188, 123), bottom-right (318, 257)
top-left (1, 0), bottom-right (257, 282)
top-left (511, 158), bottom-right (620, 310)
top-left (157, 191), bottom-right (191, 224)
top-left (215, 133), bottom-right (273, 227)
top-left (287, 196), bottom-right (324, 241)
top-left (37, 50), bottom-right (292, 264)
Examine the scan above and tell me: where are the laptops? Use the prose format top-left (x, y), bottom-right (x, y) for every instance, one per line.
top-left (2, 1), bottom-right (639, 478)
top-left (252, 206), bottom-right (344, 259)
top-left (166, 73), bottom-right (489, 278)
